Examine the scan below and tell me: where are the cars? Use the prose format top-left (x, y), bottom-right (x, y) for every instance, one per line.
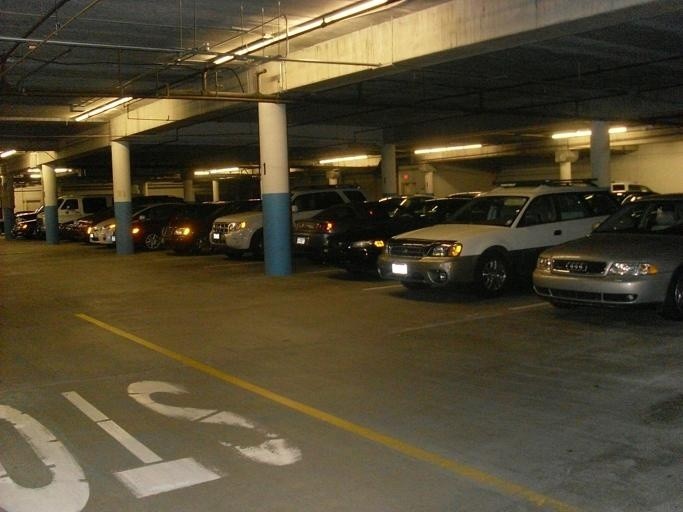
top-left (0, 194), bottom-right (262, 255)
top-left (532, 192), bottom-right (682, 323)
top-left (292, 196), bottom-right (473, 276)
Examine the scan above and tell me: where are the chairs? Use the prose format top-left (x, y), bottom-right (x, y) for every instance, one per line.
top-left (495, 201), bottom-right (598, 227)
top-left (651, 206), bottom-right (680, 231)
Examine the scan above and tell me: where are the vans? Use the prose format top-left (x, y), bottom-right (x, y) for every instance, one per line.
top-left (610, 183), bottom-right (654, 196)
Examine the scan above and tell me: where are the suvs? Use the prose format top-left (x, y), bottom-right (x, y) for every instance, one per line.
top-left (208, 185), bottom-right (368, 262)
top-left (375, 178), bottom-right (622, 300)
